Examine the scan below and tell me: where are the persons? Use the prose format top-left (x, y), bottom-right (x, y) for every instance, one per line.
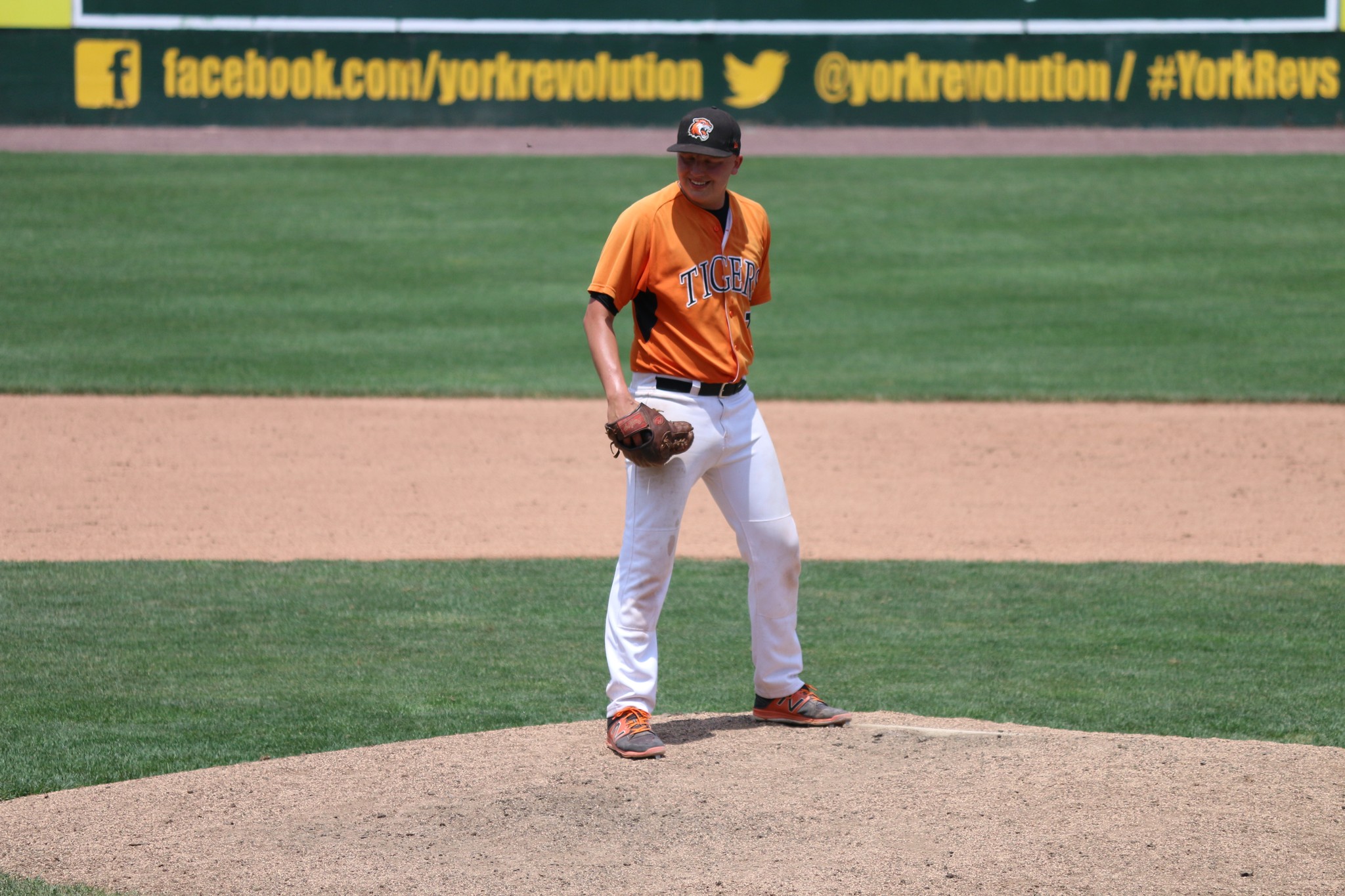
top-left (582, 110), bottom-right (852, 760)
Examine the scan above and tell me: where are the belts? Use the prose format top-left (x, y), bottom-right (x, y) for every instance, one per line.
top-left (655, 377), bottom-right (746, 398)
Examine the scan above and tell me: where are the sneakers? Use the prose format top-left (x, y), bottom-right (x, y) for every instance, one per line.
top-left (606, 706), bottom-right (666, 758)
top-left (752, 684), bottom-right (852, 725)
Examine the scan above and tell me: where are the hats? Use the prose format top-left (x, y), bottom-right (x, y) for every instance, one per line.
top-left (667, 106), bottom-right (741, 157)
